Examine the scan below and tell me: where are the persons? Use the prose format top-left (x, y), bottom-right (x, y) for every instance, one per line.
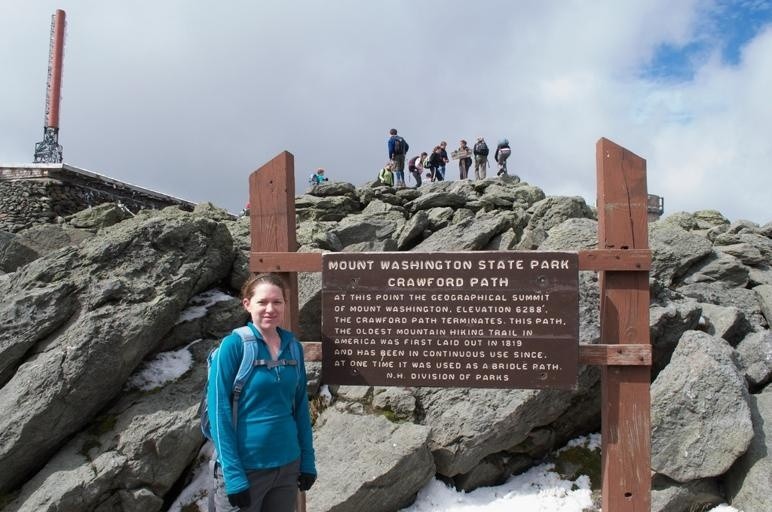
top-left (495, 138), bottom-right (511, 176)
top-left (438, 141), bottom-right (449, 180)
top-left (411, 152), bottom-right (426, 186)
top-left (430, 146), bottom-right (445, 183)
top-left (387, 128), bottom-right (408, 187)
top-left (422, 173), bottom-right (432, 182)
top-left (458, 139), bottom-right (473, 179)
top-left (377, 163), bottom-right (395, 187)
top-left (207, 273), bottom-right (317, 512)
top-left (316, 168), bottom-right (328, 183)
top-left (474, 136), bottom-right (490, 181)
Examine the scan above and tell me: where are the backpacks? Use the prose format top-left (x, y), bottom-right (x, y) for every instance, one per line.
top-left (393, 138), bottom-right (406, 156)
top-left (200, 325), bottom-right (259, 443)
top-left (477, 142), bottom-right (489, 156)
top-left (408, 156), bottom-right (420, 172)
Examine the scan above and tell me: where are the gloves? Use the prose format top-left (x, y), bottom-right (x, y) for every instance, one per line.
top-left (226, 489), bottom-right (251, 510)
top-left (297, 471), bottom-right (316, 491)
